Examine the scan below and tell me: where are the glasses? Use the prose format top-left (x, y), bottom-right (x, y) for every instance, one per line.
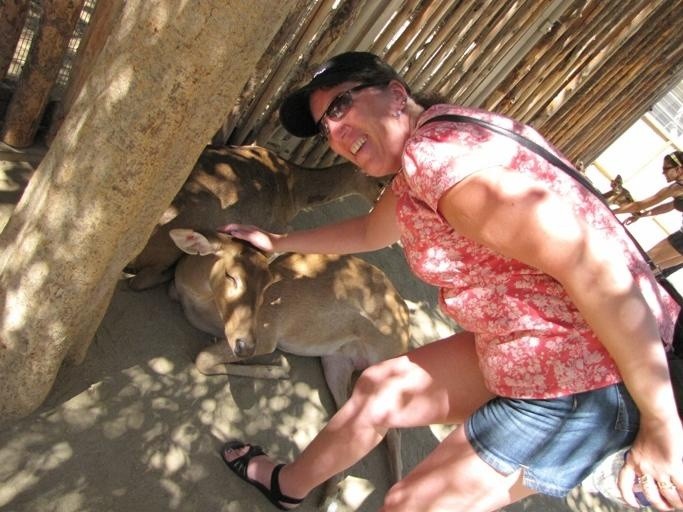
top-left (313, 80), bottom-right (371, 145)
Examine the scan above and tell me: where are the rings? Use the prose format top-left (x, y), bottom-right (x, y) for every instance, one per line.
top-left (636, 474), bottom-right (652, 485)
top-left (654, 479), bottom-right (674, 490)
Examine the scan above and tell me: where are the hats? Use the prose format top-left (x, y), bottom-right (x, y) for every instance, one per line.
top-left (274, 48), bottom-right (412, 139)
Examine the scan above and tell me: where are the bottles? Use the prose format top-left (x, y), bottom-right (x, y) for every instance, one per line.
top-left (579, 443), bottom-right (652, 511)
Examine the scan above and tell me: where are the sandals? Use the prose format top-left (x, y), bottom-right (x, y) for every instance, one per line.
top-left (217, 435), bottom-right (304, 512)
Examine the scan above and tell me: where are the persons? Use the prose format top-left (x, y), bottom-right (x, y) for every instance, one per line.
top-left (610, 149), bottom-right (682, 270)
top-left (216, 49), bottom-right (683, 512)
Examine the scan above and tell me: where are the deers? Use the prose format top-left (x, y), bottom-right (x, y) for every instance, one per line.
top-left (122, 145), bottom-right (398, 292)
top-left (168, 227), bottom-right (416, 512)
top-left (601, 175), bottom-right (641, 217)
